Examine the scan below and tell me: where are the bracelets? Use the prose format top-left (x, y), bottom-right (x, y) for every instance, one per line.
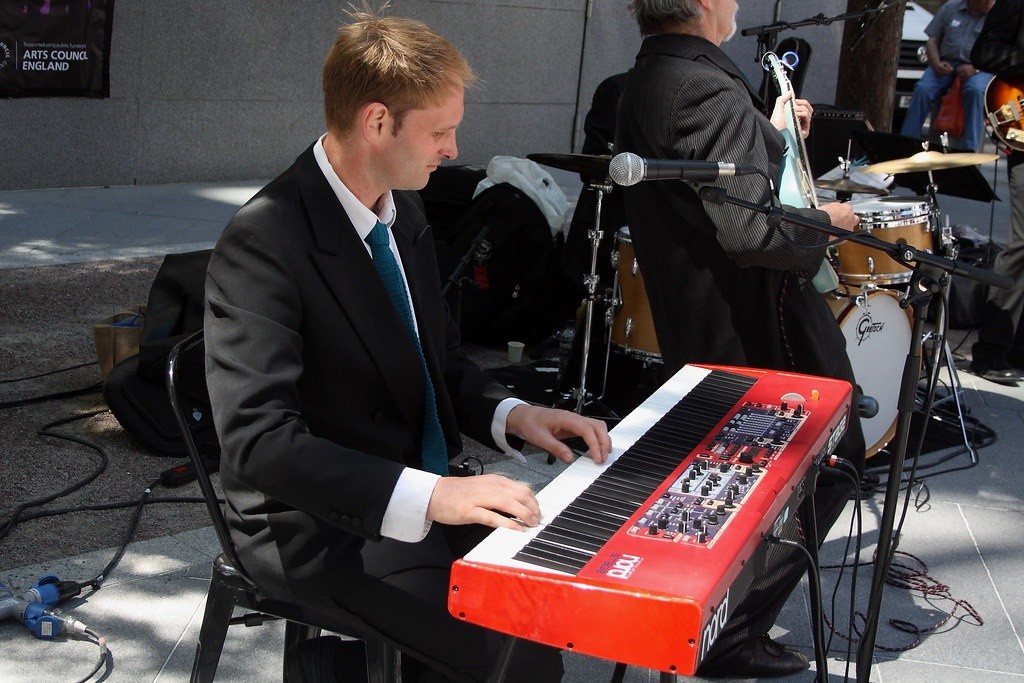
top-left (976, 69), bottom-right (980, 73)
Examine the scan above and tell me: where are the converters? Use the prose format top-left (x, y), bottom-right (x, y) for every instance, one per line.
top-left (159, 455), bottom-right (217, 488)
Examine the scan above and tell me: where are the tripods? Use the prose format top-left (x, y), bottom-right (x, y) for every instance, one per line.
top-left (919, 228), bottom-right (980, 465)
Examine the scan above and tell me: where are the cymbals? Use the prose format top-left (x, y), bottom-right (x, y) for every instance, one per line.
top-left (526, 152), bottom-right (614, 177)
top-left (856, 150), bottom-right (1002, 174)
top-left (810, 178), bottom-right (891, 195)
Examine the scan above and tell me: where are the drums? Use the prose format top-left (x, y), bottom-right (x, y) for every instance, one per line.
top-left (609, 225), bottom-right (663, 368)
top-left (819, 197), bottom-right (935, 459)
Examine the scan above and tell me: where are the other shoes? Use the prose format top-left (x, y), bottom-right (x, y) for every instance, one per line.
top-left (705, 632), bottom-right (813, 678)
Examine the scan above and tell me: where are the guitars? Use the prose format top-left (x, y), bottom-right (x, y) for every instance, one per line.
top-left (759, 52), bottom-right (841, 294)
top-left (983, 75), bottom-right (1024, 154)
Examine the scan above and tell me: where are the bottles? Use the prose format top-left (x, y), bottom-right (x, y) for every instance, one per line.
top-left (558, 320), bottom-right (577, 380)
top-left (575, 297), bottom-right (588, 330)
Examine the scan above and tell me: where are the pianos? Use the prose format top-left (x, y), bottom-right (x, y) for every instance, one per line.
top-left (445, 363), bottom-right (852, 679)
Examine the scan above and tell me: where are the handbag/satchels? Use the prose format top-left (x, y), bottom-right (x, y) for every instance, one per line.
top-left (931, 76), bottom-right (965, 136)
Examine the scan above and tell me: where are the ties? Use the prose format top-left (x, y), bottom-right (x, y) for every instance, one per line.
top-left (364, 221), bottom-right (451, 484)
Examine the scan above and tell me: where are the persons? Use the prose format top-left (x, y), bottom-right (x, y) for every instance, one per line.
top-left (580, 0), bottom-right (866, 679)
top-left (901, 0), bottom-right (996, 149)
top-left (202, 15), bottom-right (613, 683)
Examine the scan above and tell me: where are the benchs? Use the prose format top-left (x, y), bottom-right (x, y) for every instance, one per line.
top-left (896, 41), bottom-right (984, 121)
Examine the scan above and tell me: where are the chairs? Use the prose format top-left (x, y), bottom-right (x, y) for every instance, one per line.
top-left (167, 329), bottom-right (404, 683)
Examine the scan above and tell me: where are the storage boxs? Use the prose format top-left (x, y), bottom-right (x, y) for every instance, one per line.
top-left (94, 311), bottom-right (144, 376)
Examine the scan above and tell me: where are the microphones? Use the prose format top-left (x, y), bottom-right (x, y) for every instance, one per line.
top-left (609, 152), bottom-right (758, 187)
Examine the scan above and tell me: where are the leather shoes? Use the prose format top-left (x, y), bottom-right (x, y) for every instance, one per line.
top-left (970, 362), bottom-right (1021, 384)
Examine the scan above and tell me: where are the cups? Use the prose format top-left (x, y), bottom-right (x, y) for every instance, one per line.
top-left (508, 341), bottom-right (525, 363)
